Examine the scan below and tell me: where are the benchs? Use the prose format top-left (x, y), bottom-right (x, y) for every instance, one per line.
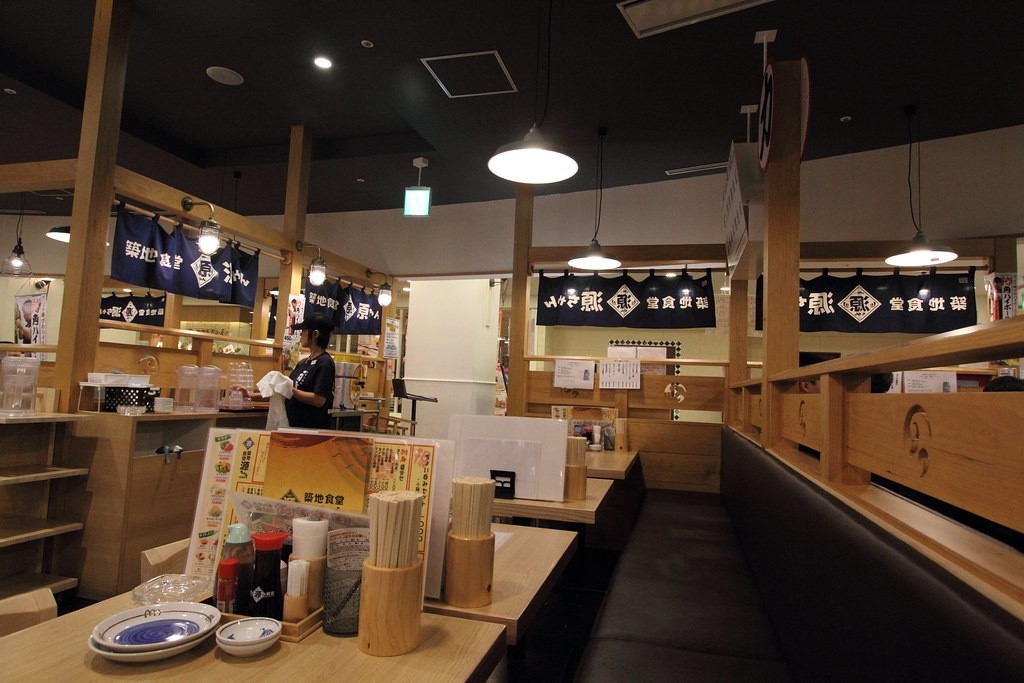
top-left (572, 424), bottom-right (1024, 683)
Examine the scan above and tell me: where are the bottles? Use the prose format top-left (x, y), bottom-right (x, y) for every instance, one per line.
top-left (211, 521), bottom-right (287, 622)
top-left (573, 427), bottom-right (593, 446)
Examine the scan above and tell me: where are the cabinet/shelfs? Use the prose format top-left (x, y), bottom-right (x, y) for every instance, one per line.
top-left (63, 409), bottom-right (267, 600)
top-left (0, 411), bottom-right (90, 599)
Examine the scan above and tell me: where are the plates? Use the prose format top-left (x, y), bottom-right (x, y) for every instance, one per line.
top-left (131, 574), bottom-right (212, 604)
top-left (88, 602), bottom-right (221, 663)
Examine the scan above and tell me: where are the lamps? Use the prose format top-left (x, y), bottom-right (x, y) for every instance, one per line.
top-left (403, 156), bottom-right (432, 218)
top-left (884, 104), bottom-right (958, 267)
top-left (1, 192), bottom-right (32, 278)
top-left (567, 125), bottom-right (621, 271)
top-left (46, 226), bottom-right (110, 247)
top-left (488, 0), bottom-right (579, 185)
top-left (180, 196), bottom-right (220, 255)
top-left (296, 240), bottom-right (327, 286)
top-left (268, 286), bottom-right (280, 295)
top-left (367, 269), bottom-right (392, 306)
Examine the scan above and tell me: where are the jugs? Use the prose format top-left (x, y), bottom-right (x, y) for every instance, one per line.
top-left (174, 364), bottom-right (230, 413)
top-left (0, 355), bottom-right (40, 417)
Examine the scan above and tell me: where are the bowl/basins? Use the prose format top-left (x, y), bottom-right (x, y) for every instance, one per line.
top-left (589, 445), bottom-right (602, 450)
top-left (215, 617), bottom-right (282, 657)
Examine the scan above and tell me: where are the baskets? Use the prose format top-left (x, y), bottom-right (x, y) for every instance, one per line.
top-left (104, 386), bottom-right (161, 412)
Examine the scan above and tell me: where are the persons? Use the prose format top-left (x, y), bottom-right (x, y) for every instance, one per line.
top-left (289, 298), bottom-right (300, 334)
top-left (228, 313), bottom-right (337, 428)
top-left (799, 353), bottom-right (1024, 553)
top-left (17, 300), bottom-right (31, 343)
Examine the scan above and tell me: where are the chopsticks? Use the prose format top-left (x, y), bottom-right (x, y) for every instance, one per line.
top-left (368, 490), bottom-right (423, 568)
top-left (450, 476), bottom-right (496, 538)
top-left (565, 437), bottom-right (587, 466)
top-left (615, 418), bottom-right (627, 434)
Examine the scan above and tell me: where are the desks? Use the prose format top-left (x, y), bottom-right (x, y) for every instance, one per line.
top-left (423, 450), bottom-right (640, 647)
top-left (0, 591), bottom-right (507, 683)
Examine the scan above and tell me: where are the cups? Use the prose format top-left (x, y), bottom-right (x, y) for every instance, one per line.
top-left (564, 465), bottom-right (587, 500)
top-left (154, 397), bottom-right (174, 413)
top-left (358, 555), bottom-right (424, 657)
top-left (614, 433), bottom-right (628, 452)
top-left (592, 434), bottom-right (600, 444)
top-left (229, 389), bottom-right (243, 407)
top-left (444, 529), bottom-right (495, 608)
top-left (283, 593), bottom-right (309, 623)
top-left (289, 553), bottom-right (327, 616)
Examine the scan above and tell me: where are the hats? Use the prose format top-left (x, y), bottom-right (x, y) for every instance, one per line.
top-left (292, 314), bottom-right (335, 332)
